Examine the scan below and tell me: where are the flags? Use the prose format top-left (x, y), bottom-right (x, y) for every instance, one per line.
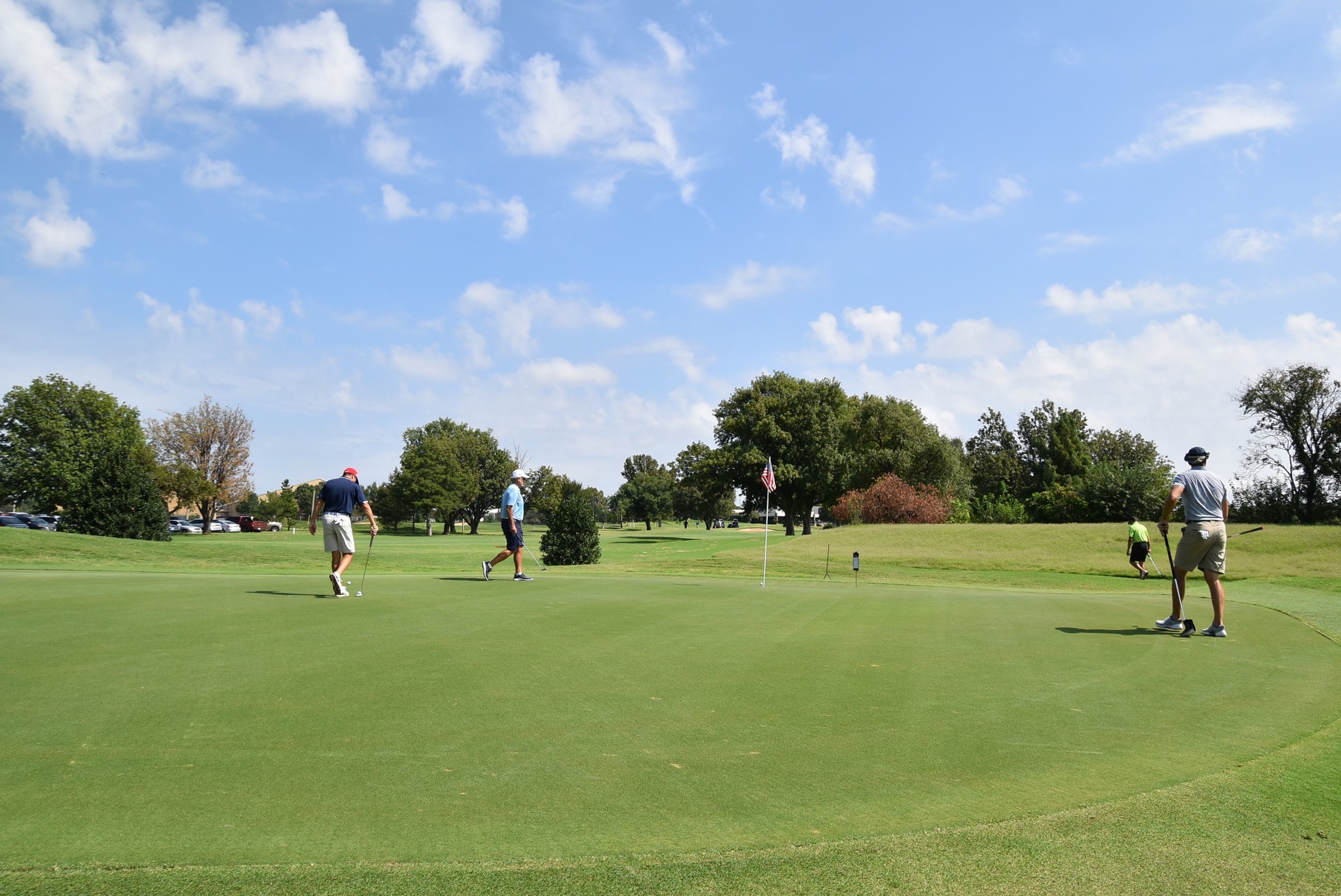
top-left (761, 462), bottom-right (777, 493)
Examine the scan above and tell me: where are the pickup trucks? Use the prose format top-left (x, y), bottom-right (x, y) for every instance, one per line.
top-left (225, 516), bottom-right (266, 532)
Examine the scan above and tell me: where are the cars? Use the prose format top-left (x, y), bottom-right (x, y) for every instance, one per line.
top-left (167, 515), bottom-right (242, 535)
top-left (0, 512), bottom-right (78, 531)
top-left (250, 516), bottom-right (283, 533)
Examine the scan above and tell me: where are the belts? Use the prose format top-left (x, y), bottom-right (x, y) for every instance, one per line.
top-left (323, 512), bottom-right (348, 516)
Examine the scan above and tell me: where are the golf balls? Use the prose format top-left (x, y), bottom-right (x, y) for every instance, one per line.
top-left (357, 591), bottom-right (361, 594)
top-left (760, 582), bottom-right (763, 585)
top-left (347, 581), bottom-right (351, 585)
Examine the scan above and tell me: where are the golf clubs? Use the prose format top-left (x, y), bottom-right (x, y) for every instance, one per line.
top-left (1161, 523), bottom-right (1197, 637)
top-left (355, 527), bottom-right (377, 597)
top-left (516, 533), bottom-right (548, 571)
top-left (1226, 526), bottom-right (1265, 538)
top-left (1147, 552), bottom-right (1164, 579)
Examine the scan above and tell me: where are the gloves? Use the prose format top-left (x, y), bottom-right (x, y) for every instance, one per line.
top-left (1199, 530), bottom-right (1209, 540)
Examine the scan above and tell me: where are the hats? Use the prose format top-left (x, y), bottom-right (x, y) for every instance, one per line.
top-left (1127, 516), bottom-right (1136, 524)
top-left (345, 467), bottom-right (359, 485)
top-left (1185, 446), bottom-right (1208, 463)
top-left (511, 469), bottom-right (530, 479)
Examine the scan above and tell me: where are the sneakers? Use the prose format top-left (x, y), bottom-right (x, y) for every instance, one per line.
top-left (482, 561), bottom-right (492, 580)
top-left (329, 571), bottom-right (343, 594)
top-left (334, 586), bottom-right (350, 597)
top-left (1201, 622), bottom-right (1226, 636)
top-left (513, 572), bottom-right (533, 581)
top-left (1141, 571), bottom-right (1148, 579)
top-left (1155, 616), bottom-right (1183, 629)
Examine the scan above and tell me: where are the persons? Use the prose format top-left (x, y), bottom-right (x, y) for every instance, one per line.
top-left (484, 470), bottom-right (533, 582)
top-left (683, 519), bottom-right (722, 529)
top-left (1155, 447), bottom-right (1229, 638)
top-left (309, 468), bottom-right (378, 597)
top-left (1126, 516), bottom-right (1151, 579)
